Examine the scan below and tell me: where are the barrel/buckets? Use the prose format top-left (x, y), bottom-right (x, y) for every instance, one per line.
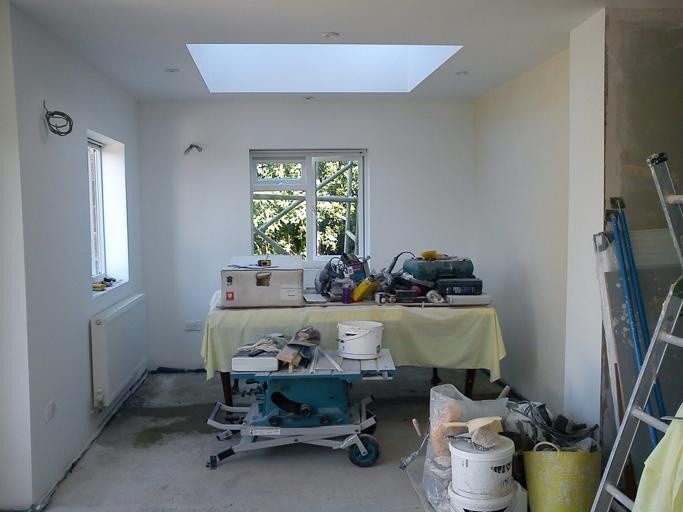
top-left (334, 319), bottom-right (384, 360)
top-left (448, 432), bottom-right (516, 499)
top-left (446, 482), bottom-right (522, 512)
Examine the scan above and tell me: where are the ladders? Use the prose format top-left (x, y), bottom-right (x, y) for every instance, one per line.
top-left (591, 272), bottom-right (682, 511)
top-left (648, 151), bottom-right (683, 270)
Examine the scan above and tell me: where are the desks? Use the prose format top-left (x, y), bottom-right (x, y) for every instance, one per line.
top-left (201, 289), bottom-right (506, 418)
